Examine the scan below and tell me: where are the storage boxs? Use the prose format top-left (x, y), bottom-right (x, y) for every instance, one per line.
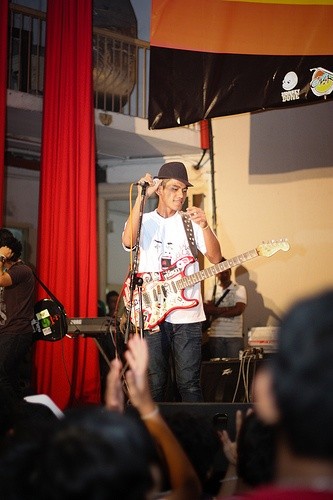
top-left (248, 327), bottom-right (280, 348)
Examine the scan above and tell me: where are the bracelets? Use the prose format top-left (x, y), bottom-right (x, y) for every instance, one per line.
top-left (141, 406), bottom-right (159, 420)
top-left (202, 225), bottom-right (209, 230)
top-left (221, 477), bottom-right (237, 482)
top-left (0, 255), bottom-right (7, 263)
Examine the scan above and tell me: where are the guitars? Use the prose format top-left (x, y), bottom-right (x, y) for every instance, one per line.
top-left (122, 239), bottom-right (291, 329)
top-left (32, 297), bottom-right (68, 342)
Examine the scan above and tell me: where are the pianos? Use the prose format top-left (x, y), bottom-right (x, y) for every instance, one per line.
top-left (66, 315), bottom-right (128, 336)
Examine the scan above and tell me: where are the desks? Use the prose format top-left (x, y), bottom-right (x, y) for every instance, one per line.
top-left (81, 332), bottom-right (239, 401)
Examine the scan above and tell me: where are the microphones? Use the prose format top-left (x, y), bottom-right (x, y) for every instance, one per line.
top-left (134, 179), bottom-right (159, 186)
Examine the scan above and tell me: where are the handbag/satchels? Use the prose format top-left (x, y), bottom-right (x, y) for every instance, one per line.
top-left (201, 320), bottom-right (210, 332)
top-left (31, 298), bottom-right (68, 341)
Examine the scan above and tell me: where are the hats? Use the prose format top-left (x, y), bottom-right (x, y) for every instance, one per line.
top-left (153, 162), bottom-right (194, 187)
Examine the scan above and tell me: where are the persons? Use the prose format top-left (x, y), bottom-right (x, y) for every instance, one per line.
top-left (122, 162), bottom-right (222, 402)
top-left (0, 227), bottom-right (333, 500)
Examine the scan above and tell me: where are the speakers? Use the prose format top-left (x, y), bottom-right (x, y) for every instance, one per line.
top-left (129, 402), bottom-right (254, 494)
top-left (200, 360), bottom-right (261, 403)
